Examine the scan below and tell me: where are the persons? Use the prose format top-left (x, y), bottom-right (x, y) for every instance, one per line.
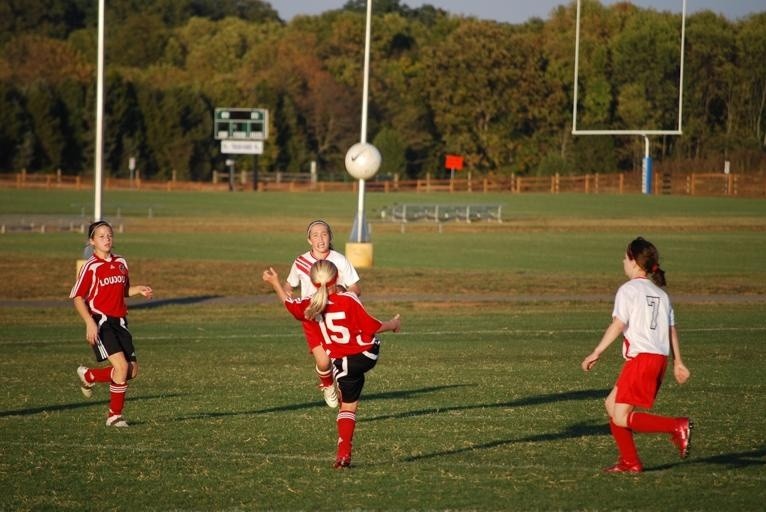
top-left (69, 221), bottom-right (152, 428)
top-left (580, 236), bottom-right (694, 475)
top-left (259, 260), bottom-right (403, 470)
top-left (281, 219), bottom-right (361, 409)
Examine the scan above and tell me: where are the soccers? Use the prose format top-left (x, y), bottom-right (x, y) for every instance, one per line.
top-left (343, 142), bottom-right (382, 181)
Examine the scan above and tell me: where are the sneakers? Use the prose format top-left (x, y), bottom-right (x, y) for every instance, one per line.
top-left (322, 383), bottom-right (338, 408)
top-left (602, 462), bottom-right (642, 472)
top-left (77, 366), bottom-right (95, 398)
top-left (106, 417), bottom-right (128, 427)
top-left (672, 416), bottom-right (693, 460)
